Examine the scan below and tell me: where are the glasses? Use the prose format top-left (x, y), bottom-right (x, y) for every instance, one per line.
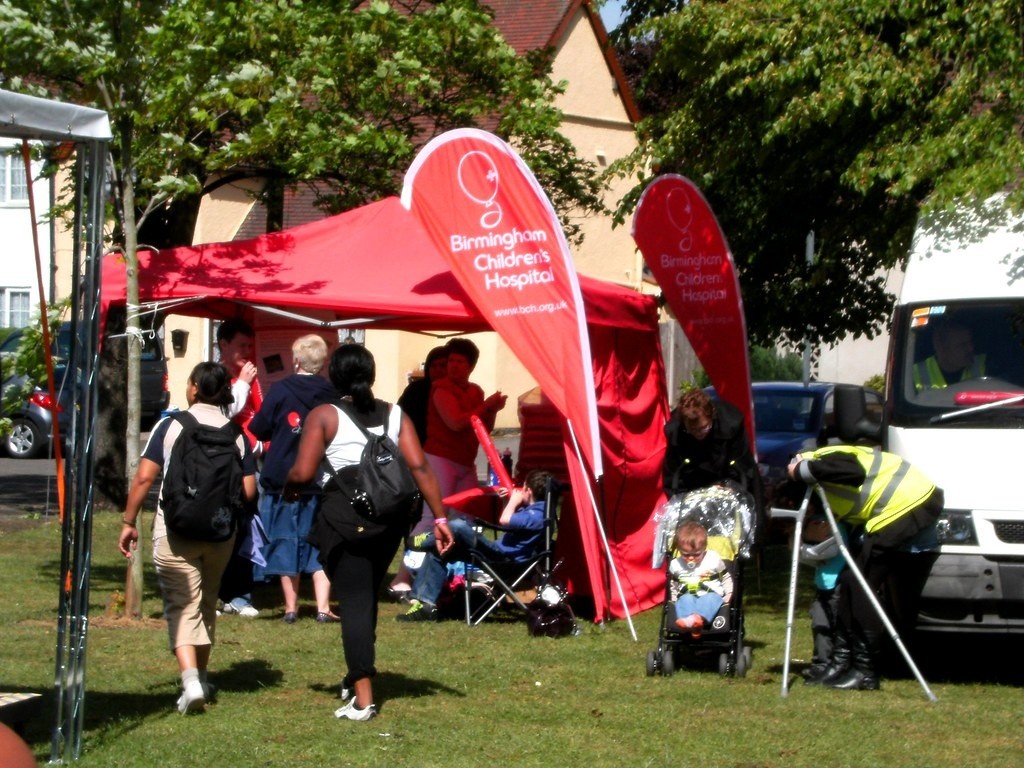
top-left (683, 419), bottom-right (712, 433)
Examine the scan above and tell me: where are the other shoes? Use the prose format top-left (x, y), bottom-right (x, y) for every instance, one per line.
top-left (318, 613), bottom-right (341, 623)
top-left (215, 610), bottom-right (221, 616)
top-left (223, 602), bottom-right (259, 617)
top-left (803, 657), bottom-right (829, 680)
top-left (283, 612), bottom-right (296, 624)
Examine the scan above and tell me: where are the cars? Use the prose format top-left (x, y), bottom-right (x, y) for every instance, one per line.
top-left (0, 320), bottom-right (170, 459)
top-left (701, 377), bottom-right (885, 550)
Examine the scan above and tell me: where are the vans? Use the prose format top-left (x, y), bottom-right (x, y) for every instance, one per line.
top-left (834, 186), bottom-right (1024, 678)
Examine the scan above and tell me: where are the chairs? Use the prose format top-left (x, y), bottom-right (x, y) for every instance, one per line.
top-left (452, 489), bottom-right (562, 627)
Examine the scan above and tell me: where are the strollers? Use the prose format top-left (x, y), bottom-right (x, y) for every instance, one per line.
top-left (646, 452), bottom-right (762, 679)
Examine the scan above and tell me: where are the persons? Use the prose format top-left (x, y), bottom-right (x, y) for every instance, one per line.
top-left (668, 521), bottom-right (734, 636)
top-left (248, 334), bottom-right (342, 622)
top-left (117, 361), bottom-right (257, 717)
top-left (288, 343), bottom-right (453, 720)
top-left (912, 319), bottom-right (994, 394)
top-left (214, 318), bottom-right (271, 618)
top-left (386, 335), bottom-right (507, 601)
top-left (662, 388), bottom-right (759, 636)
top-left (775, 446), bottom-right (945, 690)
top-left (396, 469), bottom-right (562, 624)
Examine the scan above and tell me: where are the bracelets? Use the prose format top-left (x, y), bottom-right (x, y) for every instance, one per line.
top-left (435, 518), bottom-right (447, 524)
top-left (123, 519), bottom-right (135, 526)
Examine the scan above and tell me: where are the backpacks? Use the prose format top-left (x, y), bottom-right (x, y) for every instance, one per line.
top-left (318, 400), bottom-right (425, 531)
top-left (159, 410), bottom-right (244, 545)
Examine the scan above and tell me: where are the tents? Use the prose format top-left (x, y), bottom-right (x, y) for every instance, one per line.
top-left (0, 90), bottom-right (114, 768)
top-left (98, 196), bottom-right (672, 624)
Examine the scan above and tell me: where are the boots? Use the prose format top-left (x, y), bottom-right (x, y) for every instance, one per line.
top-left (807, 647), bottom-right (855, 685)
top-left (824, 651), bottom-right (879, 690)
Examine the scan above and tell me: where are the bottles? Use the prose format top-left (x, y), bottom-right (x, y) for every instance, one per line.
top-left (487, 448), bottom-right (513, 487)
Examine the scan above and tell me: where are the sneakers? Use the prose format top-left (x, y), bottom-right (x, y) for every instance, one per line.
top-left (341, 677), bottom-right (354, 700)
top-left (407, 532), bottom-right (430, 552)
top-left (202, 683), bottom-right (211, 699)
top-left (177, 681), bottom-right (205, 717)
top-left (334, 696), bottom-right (377, 721)
top-left (395, 600), bottom-right (442, 622)
